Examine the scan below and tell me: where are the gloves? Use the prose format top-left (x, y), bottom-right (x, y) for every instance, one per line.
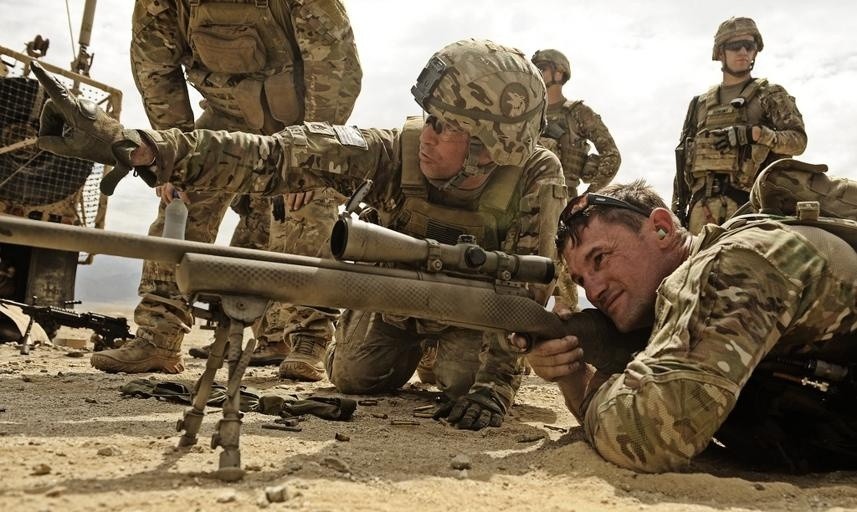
top-left (710, 124), bottom-right (755, 150)
top-left (432, 387), bottom-right (513, 432)
top-left (30, 61), bottom-right (140, 171)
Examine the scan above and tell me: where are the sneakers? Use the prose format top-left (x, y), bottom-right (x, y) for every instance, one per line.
top-left (277, 349), bottom-right (322, 383)
top-left (90, 328), bottom-right (183, 375)
top-left (248, 342), bottom-right (286, 365)
top-left (190, 340), bottom-right (235, 360)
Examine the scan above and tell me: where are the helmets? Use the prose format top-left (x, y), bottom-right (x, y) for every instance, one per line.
top-left (411, 39), bottom-right (549, 165)
top-left (531, 47), bottom-right (572, 83)
top-left (710, 17), bottom-right (764, 62)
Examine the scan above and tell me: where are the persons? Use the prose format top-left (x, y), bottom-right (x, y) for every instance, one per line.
top-left (532, 50), bottom-right (619, 352)
top-left (506, 172), bottom-right (857, 477)
top-left (18, 41), bottom-right (566, 428)
top-left (670, 14), bottom-right (805, 242)
top-left (86, 2), bottom-right (362, 385)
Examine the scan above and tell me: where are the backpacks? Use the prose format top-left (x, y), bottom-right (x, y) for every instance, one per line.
top-left (698, 157), bottom-right (856, 250)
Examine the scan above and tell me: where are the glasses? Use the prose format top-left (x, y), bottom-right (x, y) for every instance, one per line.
top-left (724, 40), bottom-right (757, 51)
top-left (553, 193), bottom-right (649, 243)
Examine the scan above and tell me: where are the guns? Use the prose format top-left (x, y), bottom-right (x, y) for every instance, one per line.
top-left (0, 199), bottom-right (636, 480)
top-left (0, 296), bottom-right (135, 355)
